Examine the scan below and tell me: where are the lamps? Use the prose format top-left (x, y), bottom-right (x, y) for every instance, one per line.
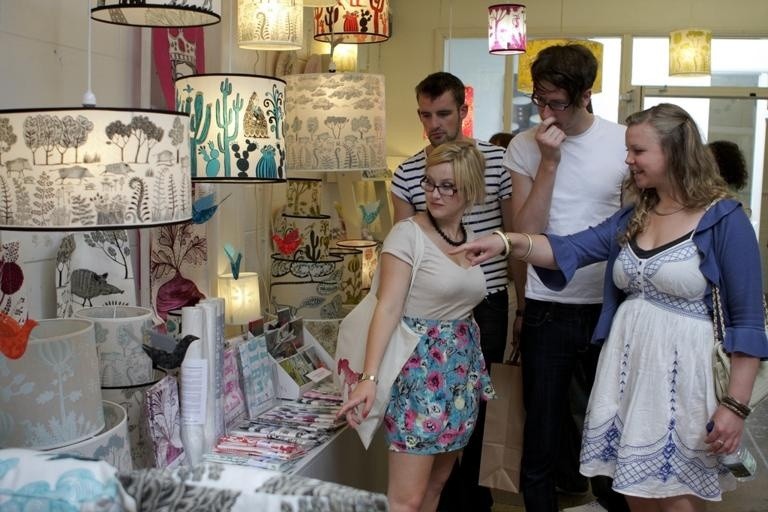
top-left (237, 1), bottom-right (306, 51)
top-left (2, 1), bottom-right (191, 230)
top-left (287, 43), bottom-right (390, 171)
top-left (88, 3), bottom-right (225, 27)
top-left (516, 1), bottom-right (602, 93)
top-left (422, 1), bottom-right (474, 140)
top-left (4, 204), bottom-right (388, 472)
top-left (361, 171), bottom-right (388, 182)
top-left (670, 28), bottom-right (711, 75)
top-left (178, 3), bottom-right (288, 183)
top-left (488, 0), bottom-right (526, 56)
top-left (314, 4), bottom-right (390, 43)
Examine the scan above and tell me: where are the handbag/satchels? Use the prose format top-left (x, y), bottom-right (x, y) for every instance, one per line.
top-left (713, 325), bottom-right (768, 412)
top-left (334, 291), bottom-right (422, 452)
top-left (477, 360), bottom-right (528, 495)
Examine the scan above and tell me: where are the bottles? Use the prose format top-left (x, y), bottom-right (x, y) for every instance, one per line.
top-left (706, 420), bottom-right (760, 482)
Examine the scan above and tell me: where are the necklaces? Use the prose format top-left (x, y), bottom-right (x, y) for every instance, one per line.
top-left (425, 206), bottom-right (468, 247)
top-left (652, 203), bottom-right (688, 217)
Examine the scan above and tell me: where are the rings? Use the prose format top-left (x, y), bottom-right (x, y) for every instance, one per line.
top-left (715, 439), bottom-right (724, 447)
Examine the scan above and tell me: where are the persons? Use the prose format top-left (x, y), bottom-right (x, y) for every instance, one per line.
top-left (501, 42), bottom-right (630, 512)
top-left (335, 138), bottom-right (500, 512)
top-left (448, 102), bottom-right (768, 512)
top-left (705, 139), bottom-right (748, 192)
top-left (488, 132), bottom-right (517, 362)
top-left (390, 70), bottom-right (528, 511)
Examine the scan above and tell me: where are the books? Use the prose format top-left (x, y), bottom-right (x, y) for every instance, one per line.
top-left (248, 307), bottom-right (329, 387)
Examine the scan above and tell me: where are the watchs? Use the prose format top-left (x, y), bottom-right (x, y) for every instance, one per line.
top-left (357, 372), bottom-right (379, 386)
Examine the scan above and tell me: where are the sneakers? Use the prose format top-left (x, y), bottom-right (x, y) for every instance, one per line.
top-left (556, 476), bottom-right (588, 497)
top-left (563, 500), bottom-right (608, 512)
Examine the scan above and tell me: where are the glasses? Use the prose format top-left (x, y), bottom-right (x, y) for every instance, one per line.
top-left (531, 88), bottom-right (577, 111)
top-left (419, 175), bottom-right (464, 197)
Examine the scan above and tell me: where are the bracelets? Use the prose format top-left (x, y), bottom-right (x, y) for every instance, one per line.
top-left (515, 232), bottom-right (533, 261)
top-left (720, 394), bottom-right (751, 419)
top-left (491, 230), bottom-right (513, 257)
